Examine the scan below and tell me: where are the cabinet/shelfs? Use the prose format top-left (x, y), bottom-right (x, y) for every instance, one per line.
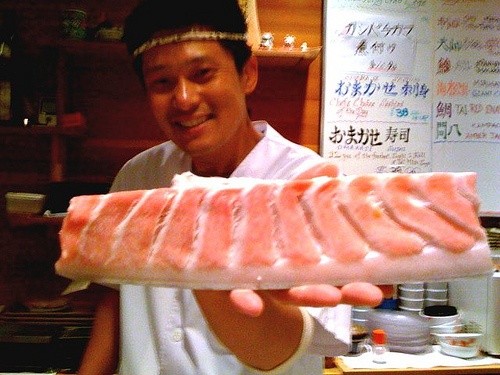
top-left (0, 31), bottom-right (322, 276)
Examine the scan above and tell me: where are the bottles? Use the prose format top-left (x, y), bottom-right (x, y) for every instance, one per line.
top-left (1, 43), bottom-right (13, 122)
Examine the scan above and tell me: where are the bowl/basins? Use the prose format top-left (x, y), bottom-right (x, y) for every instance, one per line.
top-left (96, 28), bottom-right (123, 41)
top-left (397, 282), bottom-right (485, 359)
top-left (7, 192), bottom-right (46, 215)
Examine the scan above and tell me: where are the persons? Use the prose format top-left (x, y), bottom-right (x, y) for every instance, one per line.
top-left (75, 0), bottom-right (383, 375)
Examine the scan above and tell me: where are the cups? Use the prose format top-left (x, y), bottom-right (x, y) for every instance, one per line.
top-left (60, 8), bottom-right (88, 40)
